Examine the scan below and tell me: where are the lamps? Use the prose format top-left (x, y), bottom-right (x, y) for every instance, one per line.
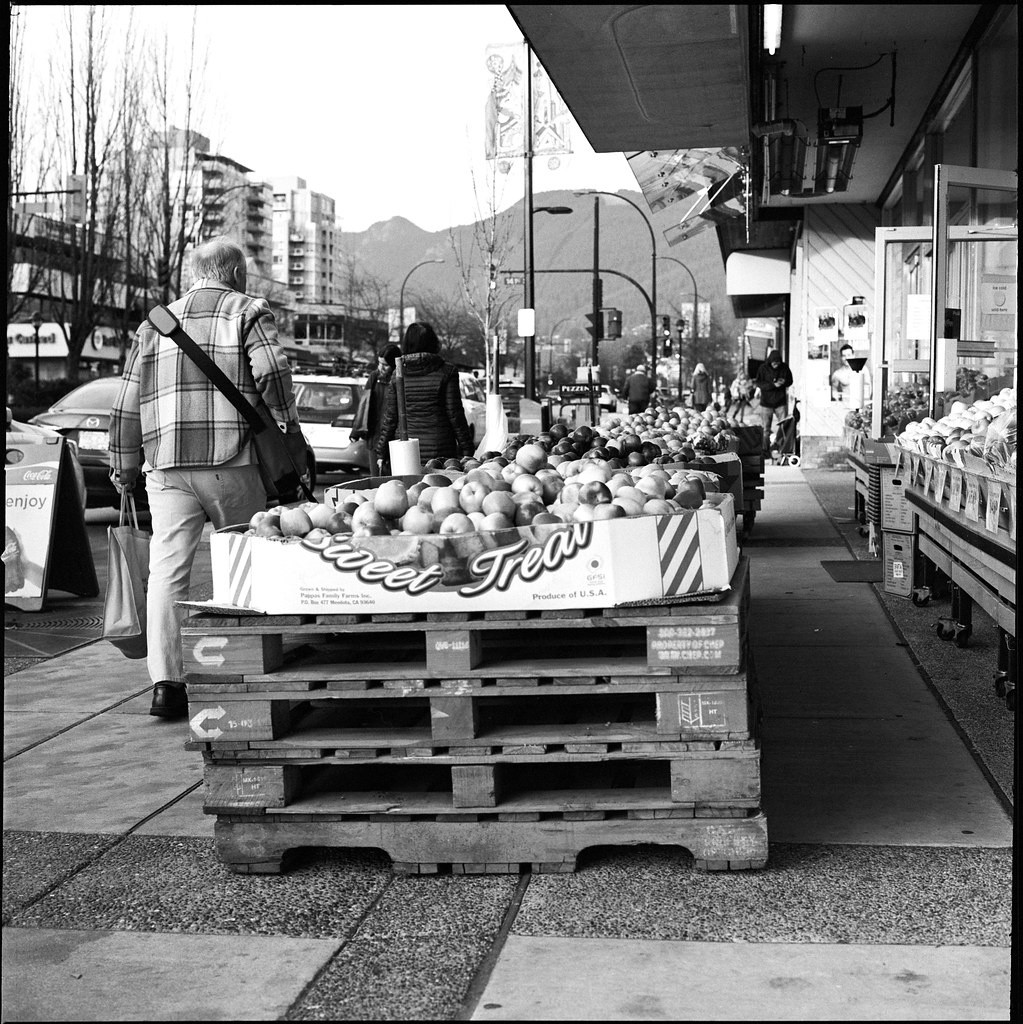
top-left (813, 106), bottom-right (863, 193)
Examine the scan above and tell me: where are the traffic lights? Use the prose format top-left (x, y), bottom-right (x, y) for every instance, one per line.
top-left (585, 312), bottom-right (603, 341)
top-left (660, 315), bottom-right (671, 338)
top-left (663, 339), bottom-right (674, 357)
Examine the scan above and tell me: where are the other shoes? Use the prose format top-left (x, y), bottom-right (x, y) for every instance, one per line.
top-left (150, 684), bottom-right (188, 718)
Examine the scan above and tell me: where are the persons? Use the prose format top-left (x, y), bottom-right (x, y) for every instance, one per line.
top-left (349, 343), bottom-right (403, 476)
top-left (377, 322), bottom-right (474, 477)
top-left (622, 364), bottom-right (656, 415)
top-left (691, 363), bottom-right (712, 412)
top-left (753, 350), bottom-right (793, 437)
top-left (107, 239), bottom-right (309, 720)
top-left (831, 344), bottom-right (871, 401)
top-left (724, 373), bottom-right (750, 423)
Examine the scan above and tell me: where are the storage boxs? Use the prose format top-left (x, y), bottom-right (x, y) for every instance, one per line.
top-left (210, 449), bottom-right (744, 615)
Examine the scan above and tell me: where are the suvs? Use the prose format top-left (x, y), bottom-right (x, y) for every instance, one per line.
top-left (290, 373), bottom-right (378, 477)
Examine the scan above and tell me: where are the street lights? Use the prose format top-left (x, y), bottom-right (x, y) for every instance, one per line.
top-left (399, 259), bottom-right (447, 353)
top-left (549, 317), bottom-right (579, 383)
top-left (524, 205), bottom-right (573, 399)
top-left (26, 311), bottom-right (44, 393)
top-left (651, 257), bottom-right (699, 369)
top-left (676, 318), bottom-right (686, 404)
top-left (572, 191), bottom-right (659, 399)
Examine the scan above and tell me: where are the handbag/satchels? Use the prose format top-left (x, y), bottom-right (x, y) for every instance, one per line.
top-left (101, 485), bottom-right (148, 659)
top-left (253, 408), bottom-right (318, 506)
top-left (353, 372), bottom-right (374, 432)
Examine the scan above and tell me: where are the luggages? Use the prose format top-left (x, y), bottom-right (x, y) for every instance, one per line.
top-left (771, 398), bottom-right (800, 467)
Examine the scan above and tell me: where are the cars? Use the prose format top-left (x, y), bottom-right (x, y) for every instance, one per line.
top-left (25, 374), bottom-right (149, 514)
top-left (456, 368), bottom-right (531, 451)
top-left (542, 383), bottom-right (620, 410)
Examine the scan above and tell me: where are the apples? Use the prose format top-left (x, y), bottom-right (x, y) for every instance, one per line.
top-left (590, 405), bottom-right (747, 445)
top-left (844, 368), bottom-right (988, 434)
top-left (895, 387), bottom-right (1017, 464)
top-left (425, 422), bottom-right (716, 472)
top-left (241, 442), bottom-right (722, 538)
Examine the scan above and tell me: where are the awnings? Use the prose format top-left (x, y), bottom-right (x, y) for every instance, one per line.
top-left (724, 249), bottom-right (792, 318)
